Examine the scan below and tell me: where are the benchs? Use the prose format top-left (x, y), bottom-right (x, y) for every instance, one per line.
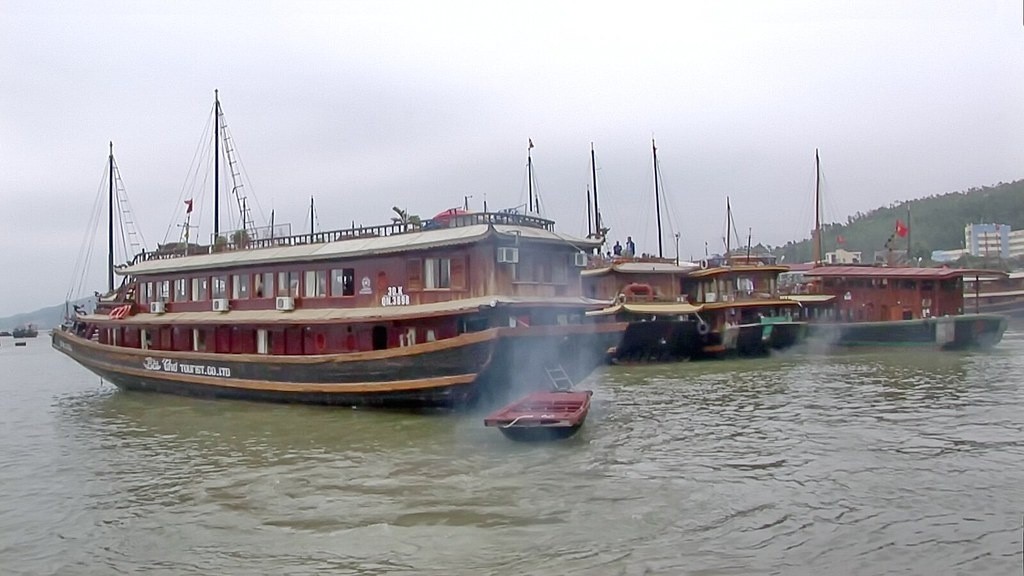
top-left (519, 403), bottom-right (579, 412)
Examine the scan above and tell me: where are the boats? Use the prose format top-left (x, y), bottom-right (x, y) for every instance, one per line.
top-left (482, 387), bottom-right (594, 442)
top-left (13, 322), bottom-right (39, 338)
top-left (48, 88), bottom-right (635, 414)
top-left (578, 137), bottom-right (1024, 369)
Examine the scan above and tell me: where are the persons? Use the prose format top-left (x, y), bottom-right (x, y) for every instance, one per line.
top-left (613, 241), bottom-right (622, 256)
top-left (626, 236), bottom-right (635, 256)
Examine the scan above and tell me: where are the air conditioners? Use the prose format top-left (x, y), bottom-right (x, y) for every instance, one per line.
top-left (213, 298), bottom-right (229, 311)
top-left (706, 293), bottom-right (716, 303)
top-left (276, 297), bottom-right (294, 310)
top-left (497, 247), bottom-right (519, 263)
top-left (151, 302), bottom-right (166, 313)
top-left (569, 253), bottom-right (588, 267)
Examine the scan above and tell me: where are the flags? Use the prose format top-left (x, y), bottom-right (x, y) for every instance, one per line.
top-left (896, 219), bottom-right (908, 239)
top-left (184, 199), bottom-right (193, 213)
